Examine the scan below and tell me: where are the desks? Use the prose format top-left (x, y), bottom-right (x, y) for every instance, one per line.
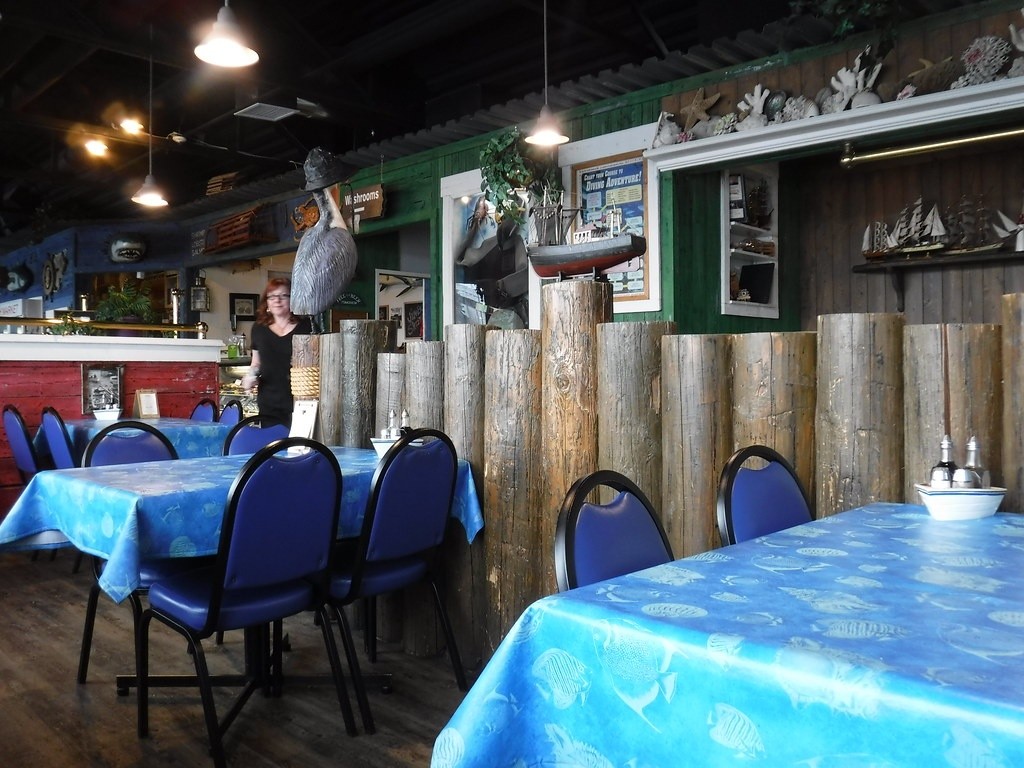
top-left (429, 504), bottom-right (1024, 768)
top-left (0, 447), bottom-right (484, 740)
top-left (31, 417), bottom-right (237, 468)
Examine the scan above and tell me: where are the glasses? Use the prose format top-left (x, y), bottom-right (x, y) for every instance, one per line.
top-left (266, 294), bottom-right (291, 300)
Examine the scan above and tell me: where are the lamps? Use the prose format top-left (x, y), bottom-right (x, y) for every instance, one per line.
top-left (525, 0), bottom-right (570, 147)
top-left (128, 25), bottom-right (169, 209)
top-left (193, 0), bottom-right (259, 68)
top-left (190, 267), bottom-right (211, 313)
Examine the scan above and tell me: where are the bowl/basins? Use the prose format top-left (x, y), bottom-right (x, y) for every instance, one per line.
top-left (371, 438), bottom-right (423, 461)
top-left (915, 482), bottom-right (1008, 520)
top-left (93, 408), bottom-right (124, 420)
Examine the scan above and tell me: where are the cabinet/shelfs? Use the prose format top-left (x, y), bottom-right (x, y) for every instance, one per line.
top-left (643, 74), bottom-right (1024, 313)
top-left (720, 162), bottom-right (780, 319)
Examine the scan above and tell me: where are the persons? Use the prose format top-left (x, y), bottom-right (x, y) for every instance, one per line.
top-left (243, 278), bottom-right (321, 430)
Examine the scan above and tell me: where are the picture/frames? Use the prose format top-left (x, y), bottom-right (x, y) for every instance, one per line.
top-left (80, 361), bottom-right (125, 416)
top-left (378, 304), bottom-right (390, 321)
top-left (402, 301), bottom-right (423, 340)
top-left (229, 292), bottom-right (260, 322)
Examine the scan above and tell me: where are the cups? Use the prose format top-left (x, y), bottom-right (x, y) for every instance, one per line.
top-left (194, 322), bottom-right (208, 339)
top-left (228, 345), bottom-right (237, 358)
top-left (237, 336), bottom-right (247, 357)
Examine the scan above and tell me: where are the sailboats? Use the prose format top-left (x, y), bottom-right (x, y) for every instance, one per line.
top-left (861, 190), bottom-right (1024, 258)
top-left (524, 185), bottom-right (648, 280)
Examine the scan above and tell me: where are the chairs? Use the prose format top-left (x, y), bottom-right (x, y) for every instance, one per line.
top-left (4, 397), bottom-right (470, 768)
top-left (553, 469), bottom-right (676, 594)
top-left (715, 445), bottom-right (816, 548)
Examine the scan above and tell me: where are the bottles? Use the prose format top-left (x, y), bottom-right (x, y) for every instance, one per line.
top-left (930, 467), bottom-right (951, 488)
top-left (380, 429), bottom-right (390, 439)
top-left (105, 403), bottom-right (118, 409)
top-left (951, 469), bottom-right (976, 488)
top-left (401, 409), bottom-right (410, 427)
top-left (389, 410), bottom-right (396, 428)
top-left (390, 429), bottom-right (402, 439)
top-left (964, 436), bottom-right (991, 488)
top-left (930, 434), bottom-right (960, 488)
top-left (108, 394), bottom-right (115, 409)
top-left (99, 395), bottom-right (106, 409)
